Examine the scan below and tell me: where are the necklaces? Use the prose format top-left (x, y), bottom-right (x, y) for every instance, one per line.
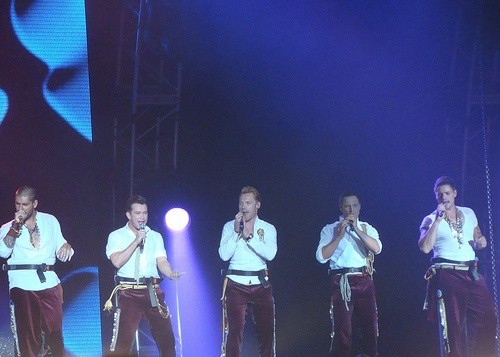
top-left (25, 223), bottom-right (37, 232)
top-left (445, 212), bottom-right (466, 246)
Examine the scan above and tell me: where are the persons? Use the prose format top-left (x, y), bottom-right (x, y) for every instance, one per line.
top-left (315, 191), bottom-right (382, 357)
top-left (105, 194), bottom-right (183, 357)
top-left (218, 185), bottom-right (276, 357)
top-left (0, 185), bottom-right (75, 357)
top-left (419, 174), bottom-right (495, 357)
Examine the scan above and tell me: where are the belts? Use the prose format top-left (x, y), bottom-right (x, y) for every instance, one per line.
top-left (223, 270), bottom-right (270, 287)
top-left (115, 276), bottom-right (163, 307)
top-left (331, 266), bottom-right (366, 274)
top-left (431, 258), bottom-right (474, 268)
top-left (5, 265), bottom-right (54, 283)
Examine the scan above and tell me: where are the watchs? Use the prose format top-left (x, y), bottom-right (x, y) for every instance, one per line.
top-left (246, 234), bottom-right (253, 241)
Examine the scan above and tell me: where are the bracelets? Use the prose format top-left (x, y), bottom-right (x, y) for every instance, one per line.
top-left (10, 226), bottom-right (19, 234)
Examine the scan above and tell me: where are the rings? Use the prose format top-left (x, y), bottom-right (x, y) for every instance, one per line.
top-left (63, 254), bottom-right (66, 257)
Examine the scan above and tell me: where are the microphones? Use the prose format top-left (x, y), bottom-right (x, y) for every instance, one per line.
top-left (240, 217), bottom-right (244, 230)
top-left (349, 220), bottom-right (354, 231)
top-left (19, 216), bottom-right (23, 224)
top-left (139, 223), bottom-right (145, 247)
top-left (438, 202), bottom-right (445, 217)
